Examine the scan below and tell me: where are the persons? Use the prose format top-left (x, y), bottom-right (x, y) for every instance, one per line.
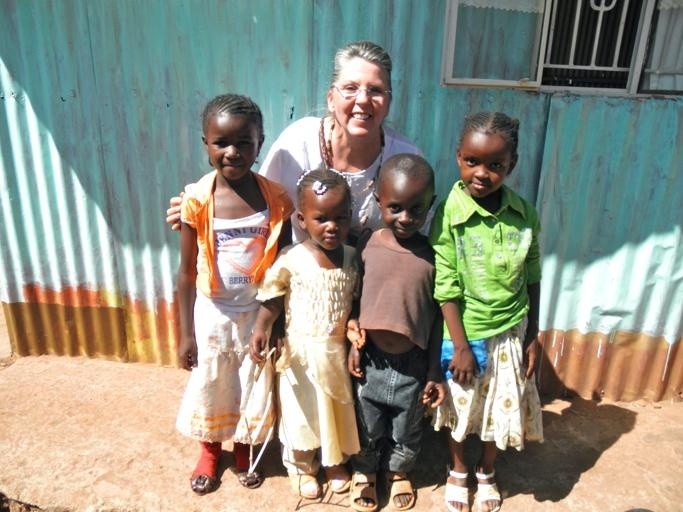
top-left (174, 94), bottom-right (296, 497)
top-left (427, 111), bottom-right (543, 512)
top-left (252, 169), bottom-right (366, 498)
top-left (166, 43), bottom-right (425, 250)
top-left (345, 152), bottom-right (446, 512)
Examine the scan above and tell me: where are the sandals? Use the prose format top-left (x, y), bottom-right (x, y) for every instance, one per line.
top-left (445, 464), bottom-right (471, 512)
top-left (351, 472), bottom-right (380, 512)
top-left (472, 458), bottom-right (502, 512)
top-left (384, 470), bottom-right (416, 511)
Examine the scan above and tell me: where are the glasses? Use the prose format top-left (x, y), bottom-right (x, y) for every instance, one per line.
top-left (333, 82), bottom-right (390, 99)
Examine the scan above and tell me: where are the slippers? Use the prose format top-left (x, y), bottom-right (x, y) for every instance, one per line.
top-left (287, 471), bottom-right (321, 499)
top-left (237, 470), bottom-right (263, 489)
top-left (190, 476), bottom-right (216, 495)
top-left (324, 466), bottom-right (351, 494)
